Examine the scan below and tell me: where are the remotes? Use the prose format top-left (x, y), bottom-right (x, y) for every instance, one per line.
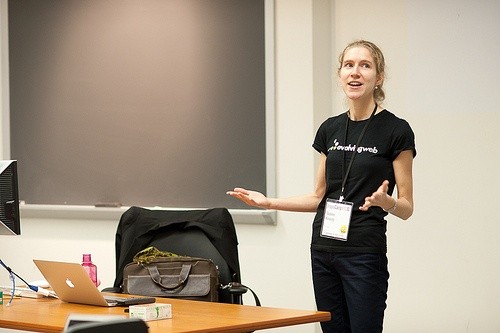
top-left (117, 298), bottom-right (156, 306)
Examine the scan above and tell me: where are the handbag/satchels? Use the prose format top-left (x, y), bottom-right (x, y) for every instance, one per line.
top-left (122, 257), bottom-right (218, 302)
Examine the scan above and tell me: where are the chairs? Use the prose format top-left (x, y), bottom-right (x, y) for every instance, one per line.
top-left (101, 207), bottom-right (247, 308)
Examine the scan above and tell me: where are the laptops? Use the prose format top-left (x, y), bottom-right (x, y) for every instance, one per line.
top-left (33, 259), bottom-right (128, 306)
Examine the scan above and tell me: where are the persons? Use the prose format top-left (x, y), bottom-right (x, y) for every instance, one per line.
top-left (226, 40), bottom-right (416, 333)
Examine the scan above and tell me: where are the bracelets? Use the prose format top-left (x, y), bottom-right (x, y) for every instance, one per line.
top-left (382, 199), bottom-right (397, 213)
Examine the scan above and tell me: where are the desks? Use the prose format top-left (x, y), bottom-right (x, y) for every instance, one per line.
top-left (0, 286), bottom-right (330, 333)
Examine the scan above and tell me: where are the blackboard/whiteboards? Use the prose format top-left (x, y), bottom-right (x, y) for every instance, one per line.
top-left (1, 2), bottom-right (278, 225)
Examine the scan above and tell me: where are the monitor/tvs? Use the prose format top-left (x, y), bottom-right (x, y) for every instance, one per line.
top-left (0, 160), bottom-right (21, 236)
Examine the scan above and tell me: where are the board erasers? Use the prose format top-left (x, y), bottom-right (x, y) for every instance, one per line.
top-left (94, 201), bottom-right (121, 207)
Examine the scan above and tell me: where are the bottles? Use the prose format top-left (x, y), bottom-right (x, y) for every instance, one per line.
top-left (81, 253), bottom-right (97, 290)
